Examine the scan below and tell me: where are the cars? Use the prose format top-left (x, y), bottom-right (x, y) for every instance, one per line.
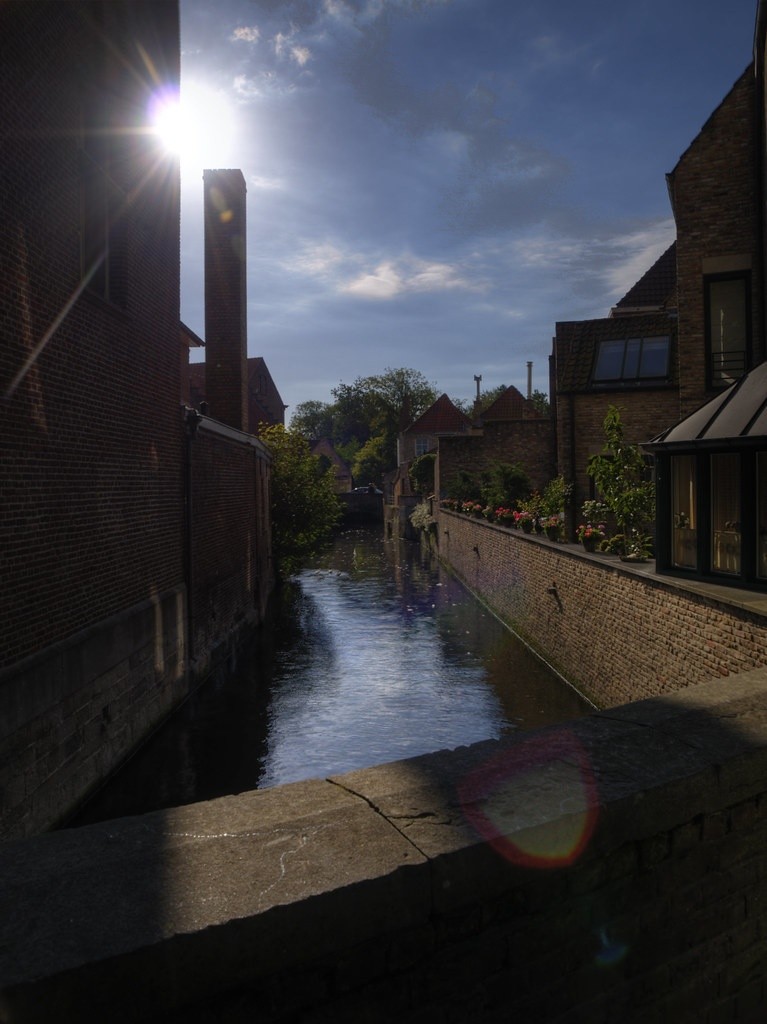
top-left (349, 487), bottom-right (384, 494)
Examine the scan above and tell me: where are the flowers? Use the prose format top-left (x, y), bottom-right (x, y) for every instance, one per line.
top-left (576, 523), bottom-right (606, 539)
top-left (440, 499), bottom-right (533, 525)
top-left (538, 514), bottom-right (565, 528)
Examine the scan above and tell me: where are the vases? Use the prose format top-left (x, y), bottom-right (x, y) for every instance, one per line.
top-left (487, 514), bottom-right (496, 523)
top-left (474, 512), bottom-right (483, 519)
top-left (523, 525), bottom-right (534, 534)
top-left (582, 538), bottom-right (598, 552)
top-left (501, 517), bottom-right (515, 527)
top-left (545, 527), bottom-right (561, 541)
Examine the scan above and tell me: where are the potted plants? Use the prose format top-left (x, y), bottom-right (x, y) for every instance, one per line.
top-left (619, 542), bottom-right (648, 562)
